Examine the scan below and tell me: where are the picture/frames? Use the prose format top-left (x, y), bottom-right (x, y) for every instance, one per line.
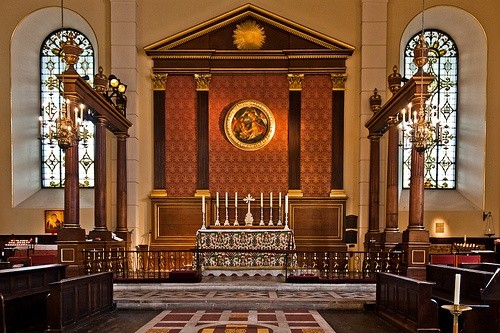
top-left (44, 210), bottom-right (65, 233)
top-left (223, 99), bottom-right (277, 152)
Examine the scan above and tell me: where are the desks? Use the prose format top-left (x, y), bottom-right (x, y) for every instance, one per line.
top-left (14, 245), bottom-right (58, 264)
top-left (196, 228), bottom-right (297, 277)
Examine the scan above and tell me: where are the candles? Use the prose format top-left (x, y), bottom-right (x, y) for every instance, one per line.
top-left (454, 274), bottom-right (462, 306)
top-left (271, 192), bottom-right (273, 205)
top-left (235, 193), bottom-right (238, 208)
top-left (285, 195), bottom-right (288, 212)
top-left (279, 191), bottom-right (282, 203)
top-left (226, 192), bottom-right (228, 206)
top-left (261, 192), bottom-right (264, 205)
top-left (216, 192), bottom-right (220, 206)
top-left (202, 196), bottom-right (205, 213)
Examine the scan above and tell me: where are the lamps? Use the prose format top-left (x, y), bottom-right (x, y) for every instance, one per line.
top-left (436, 222), bottom-right (445, 233)
top-left (103, 75), bottom-right (127, 103)
top-left (38, 0), bottom-right (95, 150)
top-left (482, 211), bottom-right (496, 239)
top-left (398, 0), bottom-right (443, 155)
